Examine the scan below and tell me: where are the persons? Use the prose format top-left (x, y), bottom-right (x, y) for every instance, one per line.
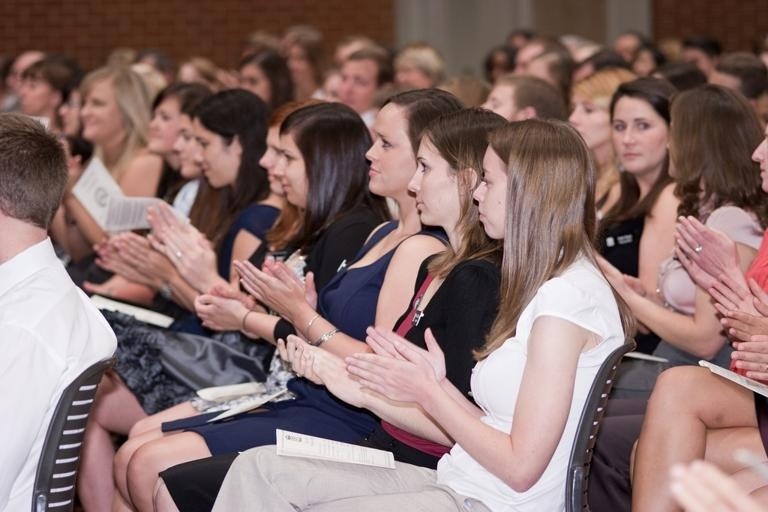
top-left (1, 29), bottom-right (767, 511)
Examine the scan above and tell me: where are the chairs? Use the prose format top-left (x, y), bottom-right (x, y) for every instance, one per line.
top-left (565, 337), bottom-right (637, 512)
top-left (31, 348), bottom-right (117, 512)
